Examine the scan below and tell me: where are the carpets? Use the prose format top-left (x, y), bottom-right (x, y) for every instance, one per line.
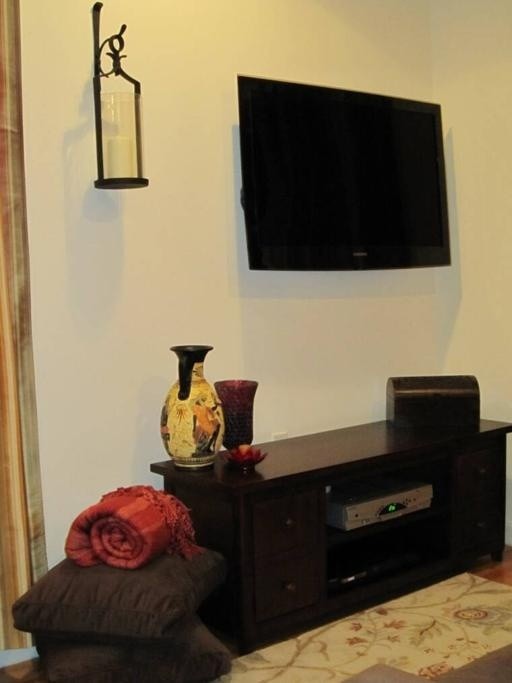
top-left (212, 572), bottom-right (512, 683)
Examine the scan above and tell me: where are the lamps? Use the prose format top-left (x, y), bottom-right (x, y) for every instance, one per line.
top-left (93, 2), bottom-right (149, 189)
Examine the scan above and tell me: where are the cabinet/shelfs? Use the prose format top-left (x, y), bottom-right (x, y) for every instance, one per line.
top-left (150, 419), bottom-right (512, 656)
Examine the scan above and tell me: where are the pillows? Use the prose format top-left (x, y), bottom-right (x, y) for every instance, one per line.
top-left (33, 611), bottom-right (231, 683)
top-left (12, 548), bottom-right (227, 647)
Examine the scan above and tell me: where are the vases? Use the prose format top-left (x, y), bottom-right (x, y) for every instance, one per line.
top-left (161, 346), bottom-right (225, 471)
top-left (214, 381), bottom-right (258, 450)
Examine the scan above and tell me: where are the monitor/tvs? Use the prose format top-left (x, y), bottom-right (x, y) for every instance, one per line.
top-left (238, 75), bottom-right (450, 271)
